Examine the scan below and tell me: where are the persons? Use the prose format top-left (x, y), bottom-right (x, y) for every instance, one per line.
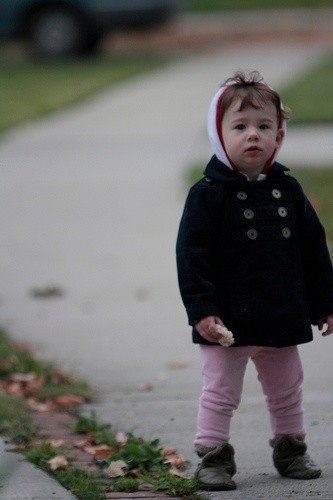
top-left (176, 75), bottom-right (333, 492)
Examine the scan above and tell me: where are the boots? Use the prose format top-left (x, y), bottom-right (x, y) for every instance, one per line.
top-left (192, 441), bottom-right (238, 490)
top-left (269, 433), bottom-right (321, 479)
